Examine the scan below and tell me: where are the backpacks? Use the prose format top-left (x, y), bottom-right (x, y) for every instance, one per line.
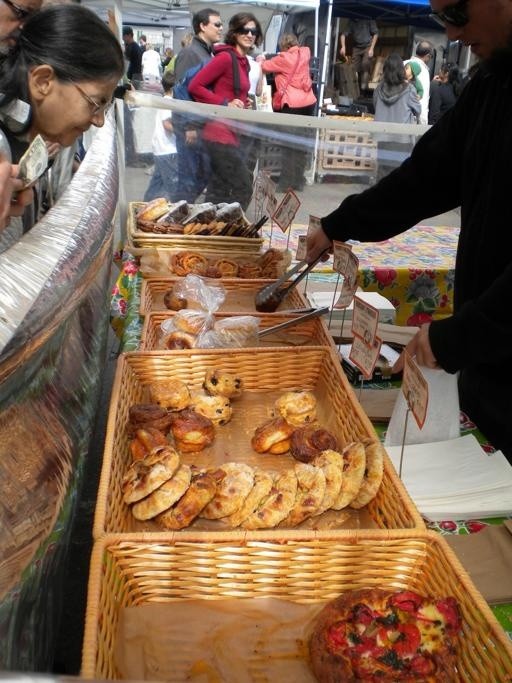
top-left (172, 52), bottom-right (239, 129)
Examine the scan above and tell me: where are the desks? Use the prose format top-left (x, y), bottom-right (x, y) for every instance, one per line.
top-left (108, 250), bottom-right (511, 642)
top-left (123, 219), bottom-right (459, 325)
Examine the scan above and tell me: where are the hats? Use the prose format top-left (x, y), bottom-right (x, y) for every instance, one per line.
top-left (411, 61), bottom-right (420, 77)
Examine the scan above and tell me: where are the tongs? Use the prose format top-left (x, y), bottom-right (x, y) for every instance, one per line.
top-left (252, 304), bottom-right (328, 340)
top-left (254, 246), bottom-right (330, 312)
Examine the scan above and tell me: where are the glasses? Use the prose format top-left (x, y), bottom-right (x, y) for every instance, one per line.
top-left (2, 0), bottom-right (33, 21)
top-left (213, 21), bottom-right (223, 28)
top-left (238, 27), bottom-right (257, 35)
top-left (70, 81), bottom-right (114, 117)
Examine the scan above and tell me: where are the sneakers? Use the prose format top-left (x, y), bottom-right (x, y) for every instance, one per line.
top-left (275, 178), bottom-right (303, 193)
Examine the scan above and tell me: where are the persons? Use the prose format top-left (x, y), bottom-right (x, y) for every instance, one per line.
top-left (339, 12), bottom-right (379, 96)
top-left (371, 54), bottom-right (425, 126)
top-left (0, 0), bottom-right (126, 231)
top-left (123, 8), bottom-right (320, 214)
top-left (429, 61), bottom-right (464, 125)
top-left (401, 41), bottom-right (430, 125)
top-left (302, 1), bottom-right (508, 470)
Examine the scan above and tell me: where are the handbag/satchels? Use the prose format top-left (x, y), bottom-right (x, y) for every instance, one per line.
top-left (271, 90), bottom-right (283, 111)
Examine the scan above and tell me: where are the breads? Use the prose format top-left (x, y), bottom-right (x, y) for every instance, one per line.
top-left (119, 439), bottom-right (384, 530)
top-left (170, 249), bottom-right (283, 280)
top-left (309, 590), bottom-right (461, 683)
top-left (127, 369), bottom-right (339, 460)
top-left (163, 290), bottom-right (187, 311)
top-left (162, 309), bottom-right (260, 350)
top-left (134, 198), bottom-right (242, 224)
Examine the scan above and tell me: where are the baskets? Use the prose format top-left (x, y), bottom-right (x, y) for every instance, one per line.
top-left (81, 201), bottom-right (510, 678)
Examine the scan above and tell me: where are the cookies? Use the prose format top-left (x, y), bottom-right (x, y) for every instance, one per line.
top-left (137, 219), bottom-right (256, 239)
